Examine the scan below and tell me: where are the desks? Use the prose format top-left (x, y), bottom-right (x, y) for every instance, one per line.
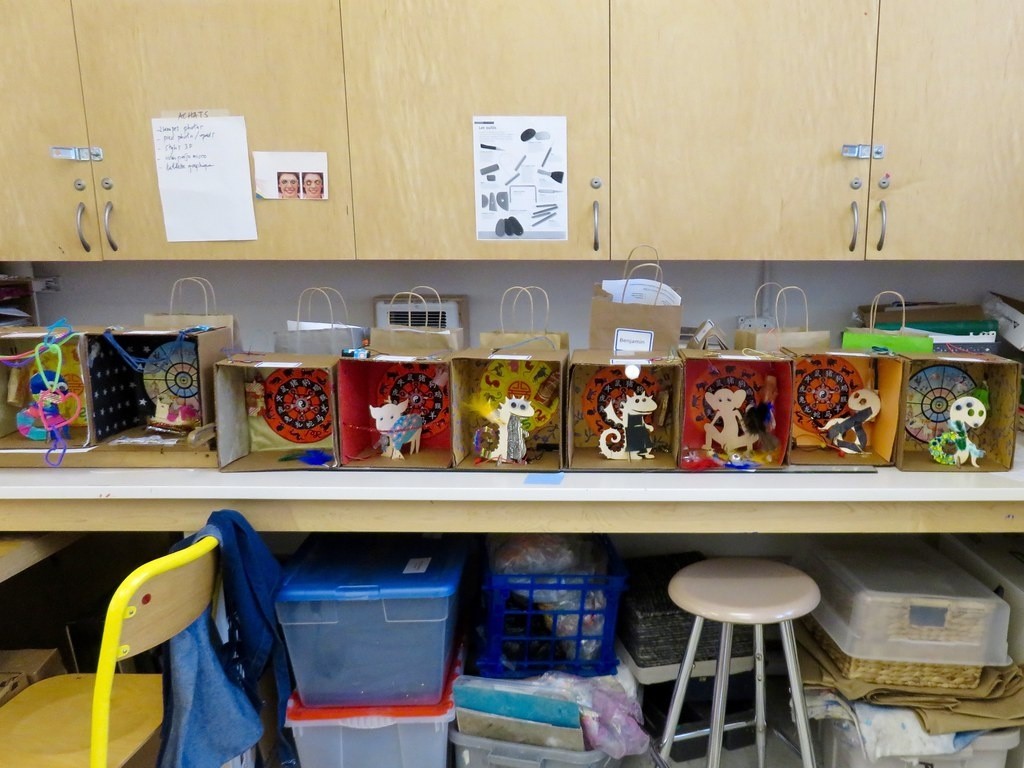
top-left (1, 432), bottom-right (1024, 535)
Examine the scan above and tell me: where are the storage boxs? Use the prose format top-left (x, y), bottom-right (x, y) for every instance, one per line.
top-left (274, 531), bottom-right (1024, 768)
top-left (0, 290), bottom-right (1024, 476)
top-left (0, 648), bottom-right (64, 714)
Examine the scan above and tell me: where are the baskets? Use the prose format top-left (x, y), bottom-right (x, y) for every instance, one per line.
top-left (805, 591), bottom-right (993, 690)
top-left (622, 551), bottom-right (755, 667)
top-left (478, 534), bottom-right (626, 679)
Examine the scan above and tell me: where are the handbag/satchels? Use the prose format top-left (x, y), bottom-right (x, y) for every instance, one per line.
top-left (143, 277), bottom-right (233, 348)
top-left (273, 287), bottom-right (365, 355)
top-left (370, 285), bottom-right (465, 356)
top-left (590, 245), bottom-right (684, 352)
top-left (735, 281), bottom-right (831, 352)
top-left (841, 290), bottom-right (934, 352)
top-left (478, 285), bottom-right (569, 352)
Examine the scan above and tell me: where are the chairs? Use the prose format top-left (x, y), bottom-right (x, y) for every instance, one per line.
top-left (0, 536), bottom-right (219, 768)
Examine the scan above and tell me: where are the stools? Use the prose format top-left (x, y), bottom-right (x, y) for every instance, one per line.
top-left (656, 557), bottom-right (818, 768)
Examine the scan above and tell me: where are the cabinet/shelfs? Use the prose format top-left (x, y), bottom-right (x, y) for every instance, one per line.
top-left (339, 1), bottom-right (611, 263)
top-left (608, 1), bottom-right (1024, 262)
top-left (0, 0), bottom-right (356, 260)
